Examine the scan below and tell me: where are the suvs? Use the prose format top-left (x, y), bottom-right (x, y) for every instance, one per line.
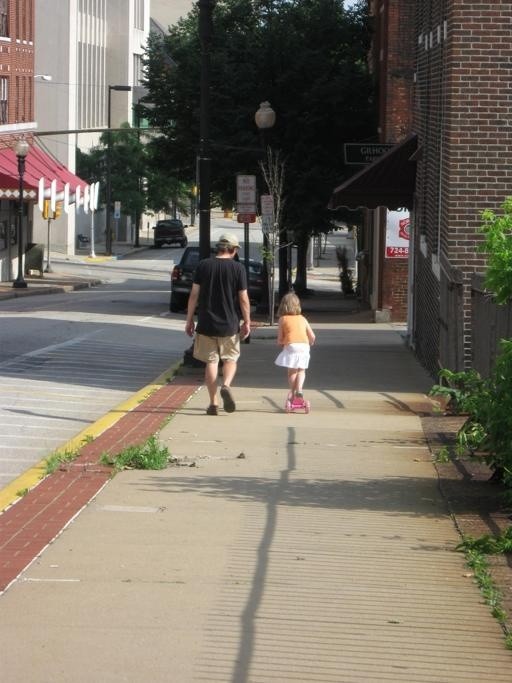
top-left (169, 245), bottom-right (241, 315)
top-left (151, 218), bottom-right (189, 250)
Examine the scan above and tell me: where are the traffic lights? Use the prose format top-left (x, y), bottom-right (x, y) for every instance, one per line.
top-left (53, 201), bottom-right (63, 219)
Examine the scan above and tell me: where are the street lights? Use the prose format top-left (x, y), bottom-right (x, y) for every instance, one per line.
top-left (14, 133), bottom-right (31, 289)
top-left (104, 83), bottom-right (134, 256)
top-left (253, 100), bottom-right (276, 324)
top-left (35, 74), bottom-right (53, 82)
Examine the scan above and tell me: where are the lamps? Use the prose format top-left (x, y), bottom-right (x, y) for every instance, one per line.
top-left (34, 71), bottom-right (53, 82)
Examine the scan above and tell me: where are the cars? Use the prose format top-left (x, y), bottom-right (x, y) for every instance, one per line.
top-left (238, 258), bottom-right (263, 300)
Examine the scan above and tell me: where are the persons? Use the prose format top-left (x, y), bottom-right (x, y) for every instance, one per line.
top-left (275, 293), bottom-right (316, 401)
top-left (185, 232), bottom-right (251, 415)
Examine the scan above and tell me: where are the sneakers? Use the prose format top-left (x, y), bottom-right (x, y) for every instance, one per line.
top-left (207, 405), bottom-right (218, 415)
top-left (220, 385), bottom-right (236, 412)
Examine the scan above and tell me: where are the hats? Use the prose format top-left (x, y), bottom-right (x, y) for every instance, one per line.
top-left (219, 234), bottom-right (242, 248)
